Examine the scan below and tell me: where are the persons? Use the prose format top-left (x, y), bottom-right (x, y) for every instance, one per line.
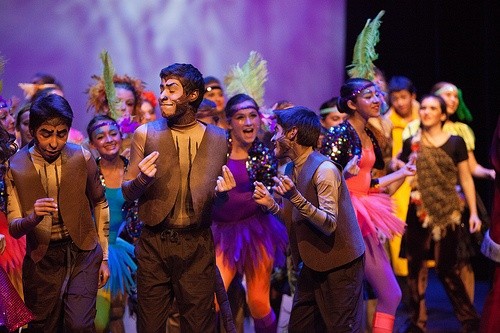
top-left (0, 11), bottom-right (500, 333)
top-left (252, 106), bottom-right (367, 333)
top-left (122, 62), bottom-right (237, 333)
top-left (3, 94), bottom-right (110, 333)
